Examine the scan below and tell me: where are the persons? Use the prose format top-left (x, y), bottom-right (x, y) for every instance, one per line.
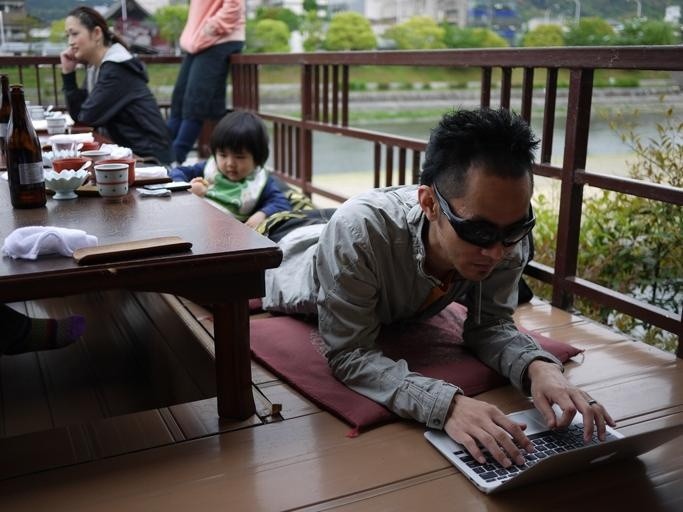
top-left (0, 301), bottom-right (86, 356)
top-left (59, 6), bottom-right (176, 172)
top-left (168, 109), bottom-right (292, 232)
top-left (166, 0), bottom-right (246, 170)
top-left (259, 106), bottom-right (616, 468)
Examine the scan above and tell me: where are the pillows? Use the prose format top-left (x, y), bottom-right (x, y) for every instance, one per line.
top-left (249, 299), bottom-right (587, 439)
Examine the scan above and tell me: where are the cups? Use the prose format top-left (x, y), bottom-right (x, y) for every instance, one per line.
top-left (24, 101), bottom-right (100, 158)
top-left (52, 157), bottom-right (91, 188)
top-left (94, 159), bottom-right (135, 188)
top-left (80, 151), bottom-right (111, 180)
top-left (93, 163), bottom-right (128, 202)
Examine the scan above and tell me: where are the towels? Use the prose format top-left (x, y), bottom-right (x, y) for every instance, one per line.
top-left (1, 222), bottom-right (101, 263)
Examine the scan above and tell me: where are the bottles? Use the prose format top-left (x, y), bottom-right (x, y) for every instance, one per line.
top-left (4, 86), bottom-right (47, 212)
top-left (0, 72), bottom-right (12, 172)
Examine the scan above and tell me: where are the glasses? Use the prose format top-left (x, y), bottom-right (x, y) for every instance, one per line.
top-left (433, 181), bottom-right (536, 248)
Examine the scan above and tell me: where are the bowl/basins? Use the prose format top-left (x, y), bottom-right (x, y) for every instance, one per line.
top-left (44, 170), bottom-right (92, 199)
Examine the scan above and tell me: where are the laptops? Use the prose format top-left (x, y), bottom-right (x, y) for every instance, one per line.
top-left (424, 403), bottom-right (683, 496)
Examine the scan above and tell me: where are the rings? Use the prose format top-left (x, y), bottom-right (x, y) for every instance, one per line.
top-left (588, 399), bottom-right (597, 405)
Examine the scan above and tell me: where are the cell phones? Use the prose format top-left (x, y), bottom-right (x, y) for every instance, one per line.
top-left (143, 181), bottom-right (192, 191)
